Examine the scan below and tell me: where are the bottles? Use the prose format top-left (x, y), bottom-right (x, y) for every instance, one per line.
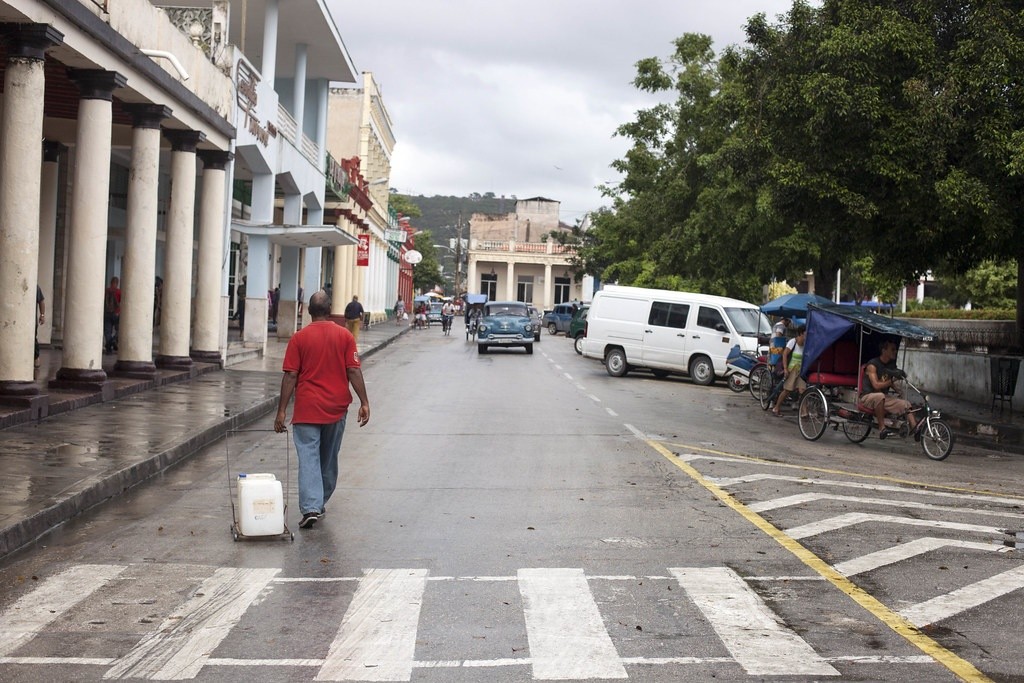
top-left (237, 473), bottom-right (276, 480)
top-left (238, 474), bottom-right (285, 536)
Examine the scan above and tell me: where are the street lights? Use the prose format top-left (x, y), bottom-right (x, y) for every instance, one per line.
top-left (433, 244), bottom-right (462, 303)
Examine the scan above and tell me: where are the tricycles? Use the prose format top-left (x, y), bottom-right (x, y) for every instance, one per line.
top-left (464, 294), bottom-right (488, 342)
top-left (798, 301), bottom-right (954, 461)
top-left (749, 294), bottom-right (840, 410)
top-left (413, 296), bottom-right (431, 329)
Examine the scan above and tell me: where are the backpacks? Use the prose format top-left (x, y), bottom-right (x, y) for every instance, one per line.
top-left (105, 288), bottom-right (121, 314)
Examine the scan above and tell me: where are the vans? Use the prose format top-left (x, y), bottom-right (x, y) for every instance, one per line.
top-left (582, 285), bottom-right (773, 385)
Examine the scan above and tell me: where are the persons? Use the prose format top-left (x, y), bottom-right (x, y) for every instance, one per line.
top-left (409, 295), bottom-right (483, 335)
top-left (767, 318), bottom-right (792, 409)
top-left (104, 276), bottom-right (121, 352)
top-left (571, 297), bottom-right (584, 320)
top-left (859, 339), bottom-right (921, 442)
top-left (394, 295), bottom-right (406, 326)
top-left (34, 284), bottom-right (45, 367)
top-left (344, 295), bottom-right (364, 342)
top-left (237, 275), bottom-right (333, 329)
top-left (274, 291), bottom-right (371, 528)
top-left (772, 324), bottom-right (809, 418)
top-left (153, 276), bottom-right (163, 327)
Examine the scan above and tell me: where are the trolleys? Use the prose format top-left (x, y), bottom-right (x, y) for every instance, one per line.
top-left (225, 429), bottom-right (295, 542)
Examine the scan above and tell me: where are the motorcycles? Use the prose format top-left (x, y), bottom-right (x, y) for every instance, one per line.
top-left (726, 344), bottom-right (768, 393)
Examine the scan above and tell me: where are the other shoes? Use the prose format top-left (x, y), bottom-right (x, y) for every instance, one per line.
top-left (34, 357), bottom-right (40, 368)
top-left (106, 348), bottom-right (112, 353)
top-left (801, 414), bottom-right (815, 420)
top-left (316, 507), bottom-right (325, 517)
top-left (880, 428), bottom-right (891, 440)
top-left (768, 401), bottom-right (774, 408)
top-left (772, 409), bottom-right (783, 418)
top-left (914, 424), bottom-right (924, 442)
top-left (298, 512), bottom-right (318, 528)
top-left (112, 341), bottom-right (118, 351)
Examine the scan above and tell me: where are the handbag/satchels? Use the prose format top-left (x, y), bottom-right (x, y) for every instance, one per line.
top-left (771, 341), bottom-right (796, 377)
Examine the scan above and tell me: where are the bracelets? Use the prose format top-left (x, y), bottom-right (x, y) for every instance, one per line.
top-left (40, 314), bottom-right (45, 317)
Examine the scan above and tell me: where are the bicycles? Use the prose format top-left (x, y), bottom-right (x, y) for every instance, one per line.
top-left (442, 313), bottom-right (454, 336)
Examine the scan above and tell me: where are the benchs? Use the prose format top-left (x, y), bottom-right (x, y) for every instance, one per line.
top-left (808, 342), bottom-right (862, 385)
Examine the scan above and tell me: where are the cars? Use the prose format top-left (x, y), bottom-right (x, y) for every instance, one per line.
top-left (543, 303), bottom-right (590, 339)
top-left (427, 302), bottom-right (444, 324)
top-left (476, 301), bottom-right (534, 354)
top-left (525, 303), bottom-right (542, 341)
